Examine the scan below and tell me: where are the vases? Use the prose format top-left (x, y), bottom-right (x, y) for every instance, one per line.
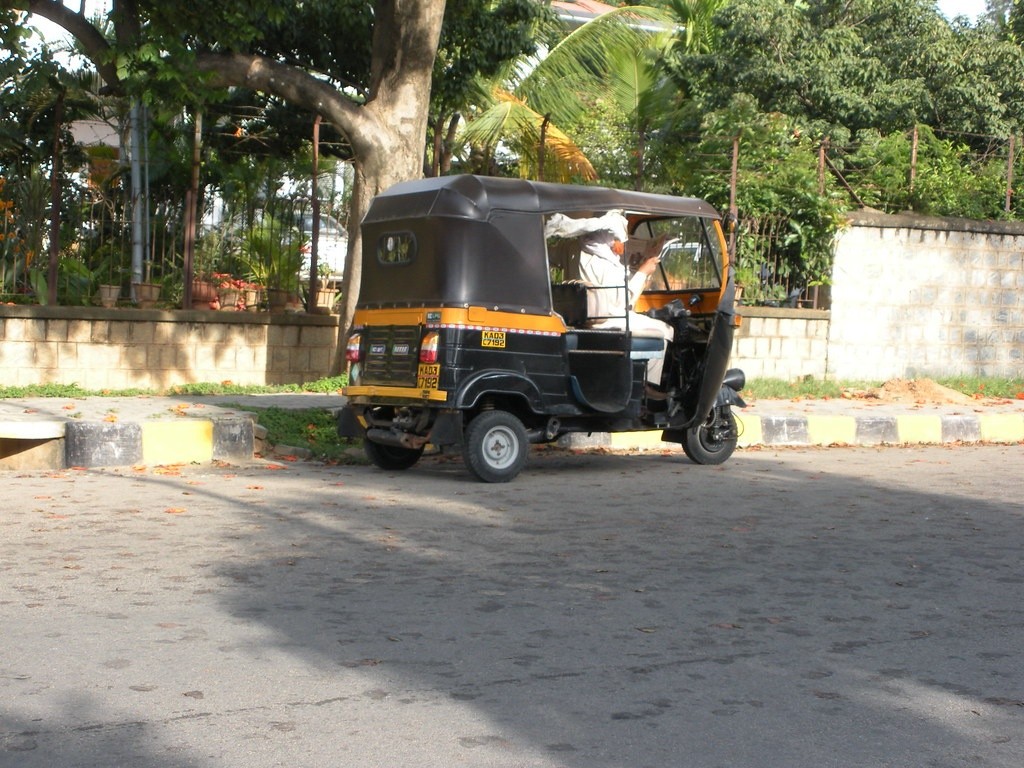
top-left (218, 289), bottom-right (239, 311)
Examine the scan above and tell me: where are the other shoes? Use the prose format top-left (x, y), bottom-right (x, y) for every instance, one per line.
top-left (642, 382), bottom-right (666, 400)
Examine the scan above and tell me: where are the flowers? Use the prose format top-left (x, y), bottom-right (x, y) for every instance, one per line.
top-left (210, 273), bottom-right (262, 311)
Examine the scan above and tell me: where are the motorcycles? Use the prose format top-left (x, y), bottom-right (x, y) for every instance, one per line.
top-left (337, 173), bottom-right (748, 484)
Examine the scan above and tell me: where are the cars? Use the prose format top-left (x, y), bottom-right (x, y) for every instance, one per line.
top-left (223, 206), bottom-right (349, 290)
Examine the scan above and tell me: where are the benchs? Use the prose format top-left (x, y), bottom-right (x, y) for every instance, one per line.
top-left (552, 284), bottom-right (665, 351)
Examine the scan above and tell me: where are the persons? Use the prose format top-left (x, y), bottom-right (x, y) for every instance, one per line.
top-left (578, 213), bottom-right (674, 399)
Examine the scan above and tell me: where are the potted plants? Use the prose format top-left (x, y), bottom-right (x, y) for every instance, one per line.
top-left (98, 205), bottom-right (340, 313)
